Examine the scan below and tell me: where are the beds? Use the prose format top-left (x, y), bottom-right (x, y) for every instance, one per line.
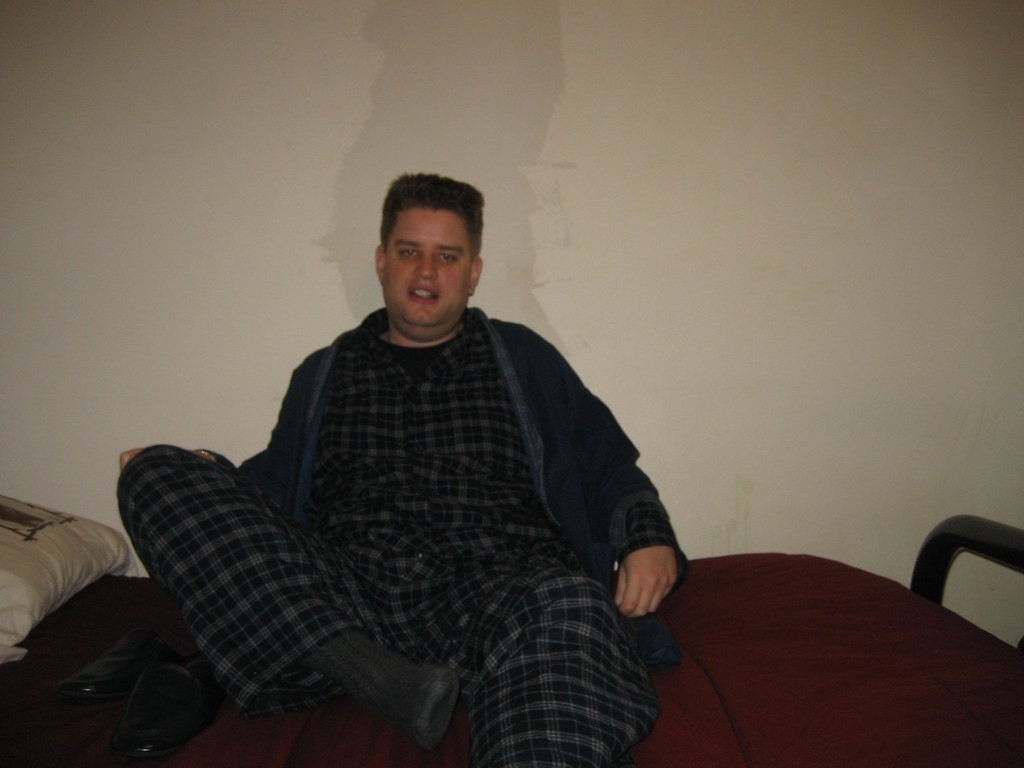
top-left (0, 517), bottom-right (1024, 768)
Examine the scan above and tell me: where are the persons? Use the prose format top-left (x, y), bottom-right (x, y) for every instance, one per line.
top-left (117, 174), bottom-right (688, 768)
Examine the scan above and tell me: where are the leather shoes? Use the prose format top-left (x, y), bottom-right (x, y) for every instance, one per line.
top-left (56, 628), bottom-right (202, 701)
top-left (109, 658), bottom-right (226, 759)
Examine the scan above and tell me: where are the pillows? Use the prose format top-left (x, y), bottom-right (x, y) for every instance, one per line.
top-left (0, 494), bottom-right (139, 664)
